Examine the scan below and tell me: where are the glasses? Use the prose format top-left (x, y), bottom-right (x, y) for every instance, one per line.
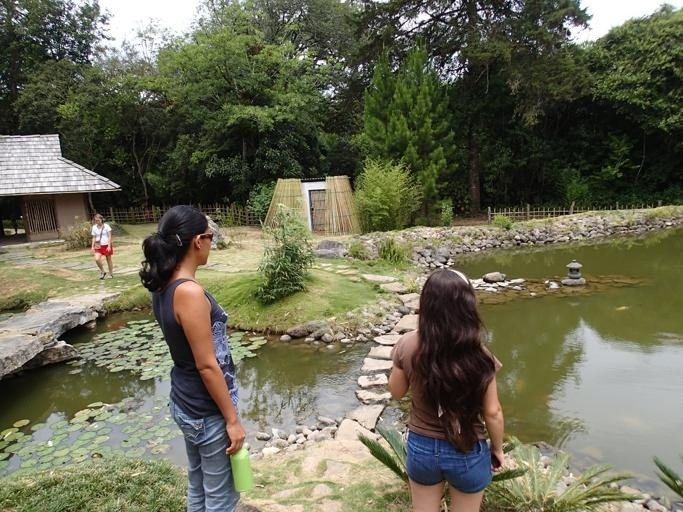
top-left (199, 230), bottom-right (213, 242)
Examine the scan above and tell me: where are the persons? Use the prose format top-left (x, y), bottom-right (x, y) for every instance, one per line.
top-left (138, 204), bottom-right (248, 512)
top-left (90, 214), bottom-right (114, 280)
top-left (389, 268), bottom-right (507, 512)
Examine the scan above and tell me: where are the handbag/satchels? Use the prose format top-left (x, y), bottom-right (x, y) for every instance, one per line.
top-left (92, 240), bottom-right (101, 249)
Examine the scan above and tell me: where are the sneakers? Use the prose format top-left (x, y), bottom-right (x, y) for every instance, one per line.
top-left (98, 271), bottom-right (112, 279)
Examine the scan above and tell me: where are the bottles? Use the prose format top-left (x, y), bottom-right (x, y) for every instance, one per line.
top-left (229, 450), bottom-right (253, 492)
